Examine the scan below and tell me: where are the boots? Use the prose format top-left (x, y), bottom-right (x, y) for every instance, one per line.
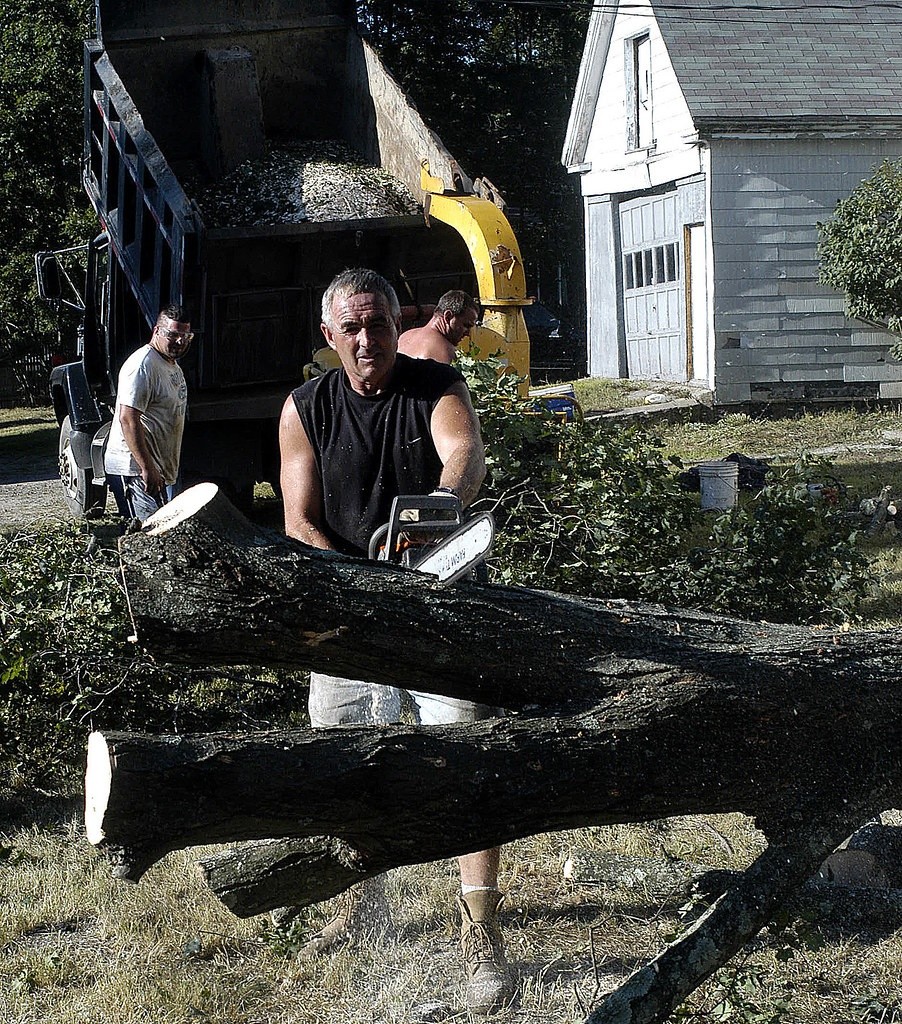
top-left (297, 872), bottom-right (391, 969)
top-left (456, 888), bottom-right (514, 1016)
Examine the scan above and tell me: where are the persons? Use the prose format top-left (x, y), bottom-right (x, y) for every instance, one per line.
top-left (277, 268), bottom-right (516, 1014)
top-left (396, 287), bottom-right (480, 365)
top-left (104, 306), bottom-right (192, 520)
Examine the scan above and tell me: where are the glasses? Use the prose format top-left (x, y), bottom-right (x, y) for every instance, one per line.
top-left (158, 326), bottom-right (194, 343)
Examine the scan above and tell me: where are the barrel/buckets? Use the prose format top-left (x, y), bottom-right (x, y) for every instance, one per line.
top-left (699, 461), bottom-right (739, 512)
top-left (529, 381), bottom-right (583, 425)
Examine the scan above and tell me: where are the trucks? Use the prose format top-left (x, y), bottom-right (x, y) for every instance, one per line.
top-left (37, 0), bottom-right (581, 542)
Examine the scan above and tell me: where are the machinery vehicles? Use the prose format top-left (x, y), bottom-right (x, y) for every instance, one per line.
top-left (305, 161), bottom-right (580, 499)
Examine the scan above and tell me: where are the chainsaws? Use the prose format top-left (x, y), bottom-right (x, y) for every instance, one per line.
top-left (366, 491), bottom-right (499, 595)
